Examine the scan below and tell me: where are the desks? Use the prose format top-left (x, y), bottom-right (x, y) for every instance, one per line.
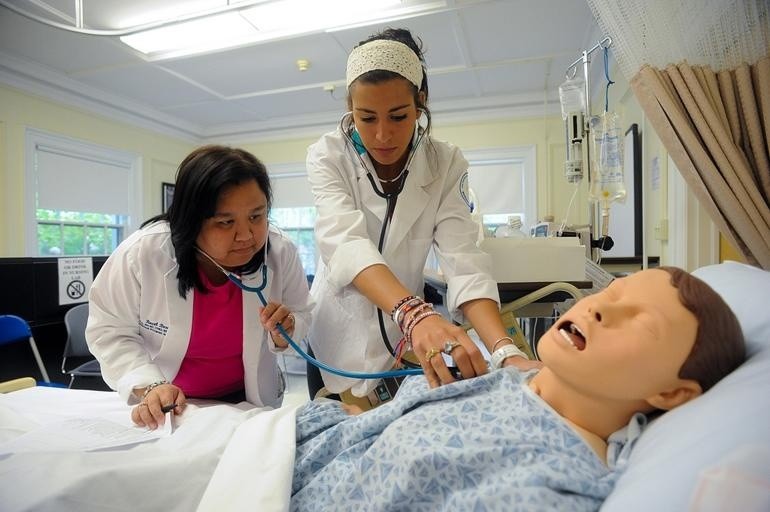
top-left (424, 268), bottom-right (592, 368)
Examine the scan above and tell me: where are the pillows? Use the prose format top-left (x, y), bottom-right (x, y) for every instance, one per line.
top-left (599, 259), bottom-right (770, 509)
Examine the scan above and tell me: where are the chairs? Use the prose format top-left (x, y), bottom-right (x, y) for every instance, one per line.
top-left (0, 316), bottom-right (66, 396)
top-left (59, 302), bottom-right (101, 387)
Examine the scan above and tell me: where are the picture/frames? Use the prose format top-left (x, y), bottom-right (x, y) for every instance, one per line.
top-left (161, 182), bottom-right (177, 213)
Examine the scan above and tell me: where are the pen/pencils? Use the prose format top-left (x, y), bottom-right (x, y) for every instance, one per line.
top-left (163, 404), bottom-right (178, 412)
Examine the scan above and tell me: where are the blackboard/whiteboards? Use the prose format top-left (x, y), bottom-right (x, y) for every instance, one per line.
top-left (595, 123), bottom-right (643, 259)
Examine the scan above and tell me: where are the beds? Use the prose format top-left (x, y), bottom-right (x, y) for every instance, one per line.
top-left (0, 279), bottom-right (586, 509)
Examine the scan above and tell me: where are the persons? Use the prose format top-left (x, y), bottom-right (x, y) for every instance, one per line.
top-left (307, 29), bottom-right (542, 406)
top-left (291, 267), bottom-right (745, 512)
top-left (85, 147), bottom-right (316, 429)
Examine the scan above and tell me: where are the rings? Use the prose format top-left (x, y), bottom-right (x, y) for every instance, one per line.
top-left (138, 403), bottom-right (148, 407)
top-left (425, 348), bottom-right (440, 362)
top-left (444, 340), bottom-right (462, 355)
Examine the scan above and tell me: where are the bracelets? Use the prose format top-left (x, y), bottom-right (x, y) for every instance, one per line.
top-left (391, 295), bottom-right (442, 343)
top-left (491, 337), bottom-right (529, 369)
top-left (141, 380), bottom-right (170, 399)
